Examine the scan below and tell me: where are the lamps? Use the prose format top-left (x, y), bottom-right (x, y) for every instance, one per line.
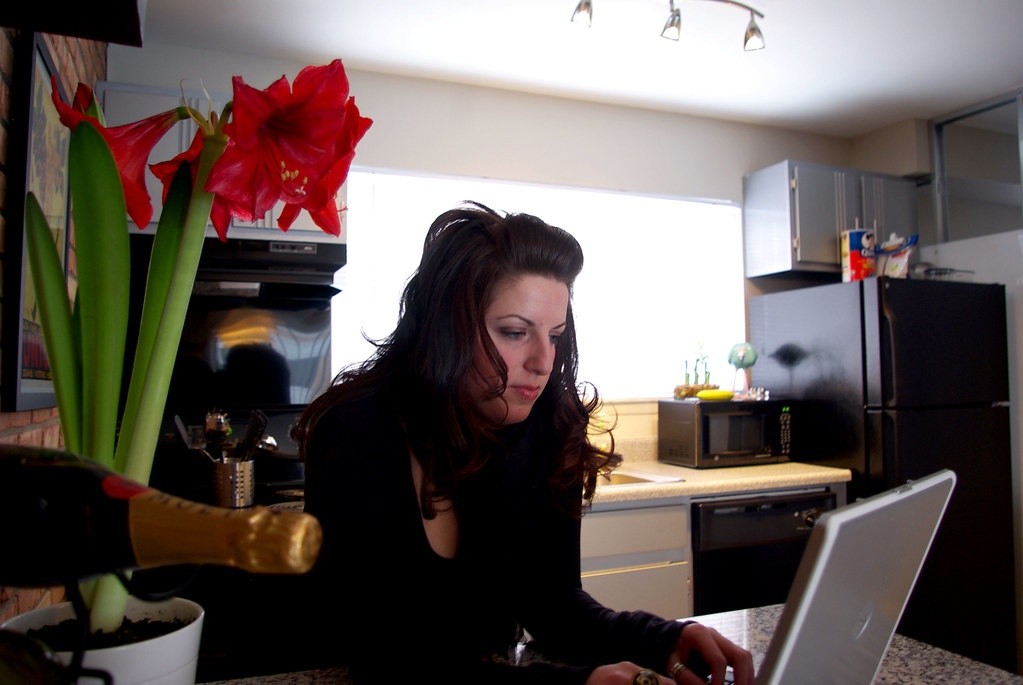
top-left (570, 0), bottom-right (594, 29)
top-left (743, 10), bottom-right (766, 51)
top-left (660, 0), bottom-right (682, 41)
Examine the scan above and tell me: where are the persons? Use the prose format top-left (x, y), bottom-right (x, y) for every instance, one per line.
top-left (292, 204), bottom-right (756, 685)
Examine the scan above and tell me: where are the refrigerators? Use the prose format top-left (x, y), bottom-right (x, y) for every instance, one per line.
top-left (750, 284), bottom-right (1011, 619)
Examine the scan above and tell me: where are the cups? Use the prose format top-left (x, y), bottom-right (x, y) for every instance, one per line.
top-left (216, 458), bottom-right (255, 507)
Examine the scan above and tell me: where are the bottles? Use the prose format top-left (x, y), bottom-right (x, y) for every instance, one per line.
top-left (1, 443), bottom-right (321, 588)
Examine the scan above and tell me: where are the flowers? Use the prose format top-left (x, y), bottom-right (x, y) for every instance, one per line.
top-left (28, 58), bottom-right (372, 624)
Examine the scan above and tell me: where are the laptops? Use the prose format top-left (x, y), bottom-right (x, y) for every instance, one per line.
top-left (698, 470), bottom-right (956, 685)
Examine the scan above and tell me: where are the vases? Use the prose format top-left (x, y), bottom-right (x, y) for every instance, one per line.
top-left (0, 595), bottom-right (205, 685)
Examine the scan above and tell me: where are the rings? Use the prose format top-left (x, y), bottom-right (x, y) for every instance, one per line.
top-left (670, 662), bottom-right (686, 677)
top-left (632, 670), bottom-right (660, 685)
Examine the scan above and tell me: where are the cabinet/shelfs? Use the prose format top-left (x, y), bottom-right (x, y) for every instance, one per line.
top-left (743, 159), bottom-right (919, 277)
top-left (581, 495), bottom-right (691, 620)
top-left (92, 81), bottom-right (349, 247)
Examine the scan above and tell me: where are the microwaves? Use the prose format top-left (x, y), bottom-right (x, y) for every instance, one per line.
top-left (658, 394), bottom-right (800, 468)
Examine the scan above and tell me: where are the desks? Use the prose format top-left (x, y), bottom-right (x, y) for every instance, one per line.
top-left (674, 602), bottom-right (1023, 685)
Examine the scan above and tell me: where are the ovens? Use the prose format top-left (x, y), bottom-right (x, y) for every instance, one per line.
top-left (689, 485), bottom-right (837, 623)
